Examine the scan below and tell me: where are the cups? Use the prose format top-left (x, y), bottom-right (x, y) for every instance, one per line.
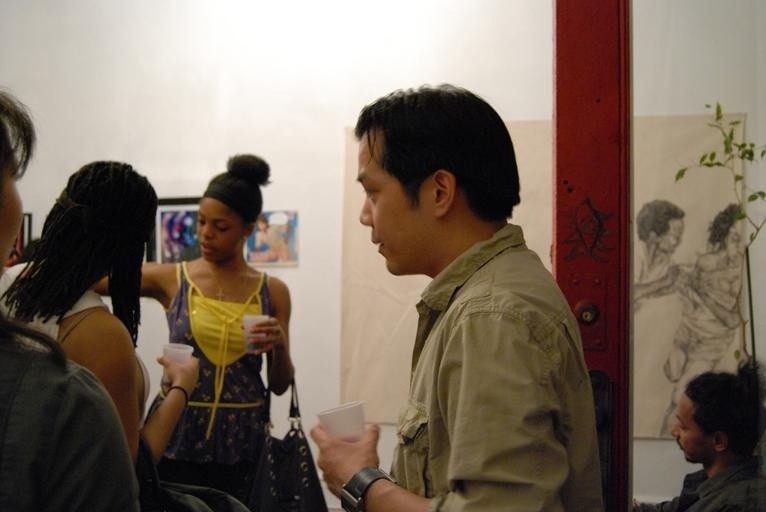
top-left (316, 400), bottom-right (366, 445)
top-left (242, 314), bottom-right (270, 355)
top-left (159, 343), bottom-right (196, 387)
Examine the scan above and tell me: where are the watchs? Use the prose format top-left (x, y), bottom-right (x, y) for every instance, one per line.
top-left (340, 466), bottom-right (396, 512)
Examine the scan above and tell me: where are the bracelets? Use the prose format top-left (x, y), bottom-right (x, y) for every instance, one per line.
top-left (166, 384), bottom-right (192, 408)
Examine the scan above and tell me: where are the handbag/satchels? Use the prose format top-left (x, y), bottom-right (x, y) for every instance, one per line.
top-left (243, 377), bottom-right (329, 511)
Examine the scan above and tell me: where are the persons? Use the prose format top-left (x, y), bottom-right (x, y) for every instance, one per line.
top-left (659, 202), bottom-right (745, 439)
top-left (633, 359), bottom-right (766, 512)
top-left (0, 89), bottom-right (141, 511)
top-left (88, 147), bottom-right (295, 511)
top-left (310, 82), bottom-right (604, 512)
top-left (631, 199), bottom-right (686, 314)
top-left (0, 158), bottom-right (200, 486)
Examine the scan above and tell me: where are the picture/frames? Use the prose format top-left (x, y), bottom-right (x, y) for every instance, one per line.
top-left (243, 206), bottom-right (302, 270)
top-left (5, 213), bottom-right (32, 266)
top-left (144, 196), bottom-right (202, 264)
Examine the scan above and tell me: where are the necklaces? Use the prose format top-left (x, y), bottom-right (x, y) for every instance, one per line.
top-left (204, 260), bottom-right (244, 303)
